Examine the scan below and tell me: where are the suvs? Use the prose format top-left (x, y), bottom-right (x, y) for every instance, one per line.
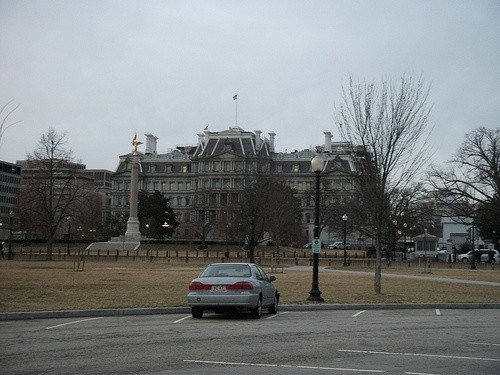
top-left (456, 248), bottom-right (500, 265)
top-left (327, 241), bottom-right (352, 250)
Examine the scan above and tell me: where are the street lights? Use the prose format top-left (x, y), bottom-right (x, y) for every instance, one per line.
top-left (5, 210), bottom-right (15, 260)
top-left (392, 218), bottom-right (398, 262)
top-left (305, 149), bottom-right (325, 301)
top-left (66, 215), bottom-right (72, 256)
top-left (342, 213), bottom-right (348, 266)
top-left (403, 222), bottom-right (408, 261)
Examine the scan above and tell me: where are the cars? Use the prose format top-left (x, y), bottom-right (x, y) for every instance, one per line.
top-left (185, 262), bottom-right (281, 319)
top-left (288, 240), bottom-right (324, 250)
